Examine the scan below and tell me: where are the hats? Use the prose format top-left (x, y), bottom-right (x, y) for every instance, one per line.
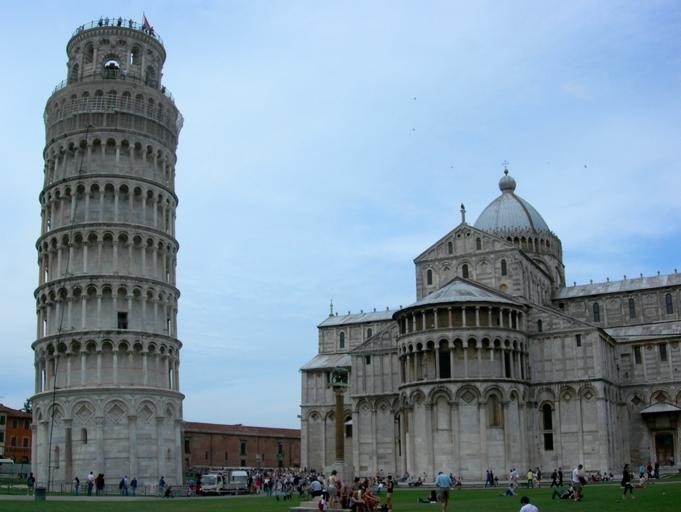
top-left (0, 459), bottom-right (14, 465)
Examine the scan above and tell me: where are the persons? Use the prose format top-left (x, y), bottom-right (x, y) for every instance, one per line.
top-left (98, 15), bottom-right (156, 38)
